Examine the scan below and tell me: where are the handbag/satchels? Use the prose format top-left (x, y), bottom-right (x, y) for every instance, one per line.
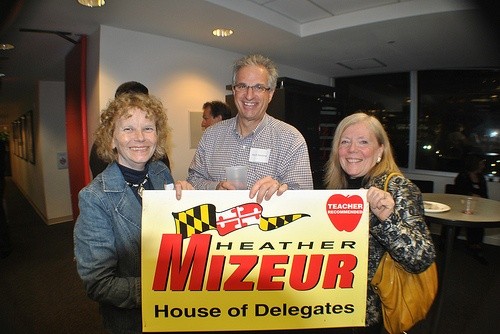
top-left (371, 173), bottom-right (437, 334)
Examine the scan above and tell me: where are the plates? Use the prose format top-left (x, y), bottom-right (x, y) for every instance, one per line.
top-left (423, 200), bottom-right (451, 212)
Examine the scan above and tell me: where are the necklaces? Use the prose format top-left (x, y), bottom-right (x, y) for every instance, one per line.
top-left (122, 174), bottom-right (151, 198)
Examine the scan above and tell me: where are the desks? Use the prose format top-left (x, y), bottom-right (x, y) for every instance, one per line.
top-left (420, 193), bottom-right (500, 334)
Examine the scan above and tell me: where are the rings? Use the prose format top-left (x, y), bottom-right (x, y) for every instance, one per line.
top-left (383, 191), bottom-right (388, 199)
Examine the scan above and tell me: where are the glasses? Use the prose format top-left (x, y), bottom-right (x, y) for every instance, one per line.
top-left (233, 83), bottom-right (270, 93)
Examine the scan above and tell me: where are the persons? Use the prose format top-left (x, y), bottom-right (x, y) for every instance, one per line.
top-left (325, 113), bottom-right (438, 334)
top-left (187, 54), bottom-right (315, 203)
top-left (443, 149), bottom-right (491, 265)
top-left (74, 91), bottom-right (194, 334)
top-left (89, 81), bottom-right (171, 182)
top-left (201, 100), bottom-right (233, 129)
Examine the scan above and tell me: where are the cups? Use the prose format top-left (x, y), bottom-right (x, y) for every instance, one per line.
top-left (461, 196), bottom-right (476, 214)
top-left (225, 166), bottom-right (248, 190)
